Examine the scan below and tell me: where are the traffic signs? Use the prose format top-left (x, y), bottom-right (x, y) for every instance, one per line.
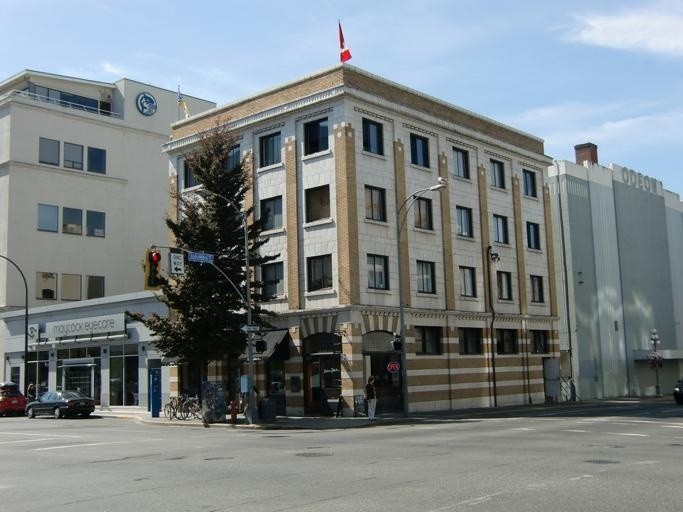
top-left (239, 322), bottom-right (261, 333)
top-left (188, 251), bottom-right (214, 263)
top-left (170, 252), bottom-right (184, 274)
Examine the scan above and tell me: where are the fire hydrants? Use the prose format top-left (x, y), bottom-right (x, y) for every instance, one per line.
top-left (229, 400), bottom-right (239, 424)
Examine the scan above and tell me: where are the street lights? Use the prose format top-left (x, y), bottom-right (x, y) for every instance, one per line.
top-left (398, 175), bottom-right (447, 417)
top-left (650, 329), bottom-right (663, 397)
top-left (149, 188), bottom-right (261, 423)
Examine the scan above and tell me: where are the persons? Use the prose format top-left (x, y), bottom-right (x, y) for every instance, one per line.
top-left (366, 376), bottom-right (377, 420)
top-left (27, 384), bottom-right (36, 402)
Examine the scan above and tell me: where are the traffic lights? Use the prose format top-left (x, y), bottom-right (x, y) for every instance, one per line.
top-left (387, 360), bottom-right (400, 373)
top-left (146, 248), bottom-right (161, 290)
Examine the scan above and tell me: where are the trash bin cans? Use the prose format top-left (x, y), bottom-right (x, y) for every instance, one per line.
top-left (260, 399), bottom-right (275, 423)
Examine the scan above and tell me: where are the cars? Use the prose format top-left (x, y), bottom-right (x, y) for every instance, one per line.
top-left (25, 388), bottom-right (99, 419)
top-left (0, 381), bottom-right (28, 417)
top-left (673, 380), bottom-right (683, 404)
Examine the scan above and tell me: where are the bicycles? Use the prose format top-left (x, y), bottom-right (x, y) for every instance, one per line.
top-left (163, 394), bottom-right (204, 421)
